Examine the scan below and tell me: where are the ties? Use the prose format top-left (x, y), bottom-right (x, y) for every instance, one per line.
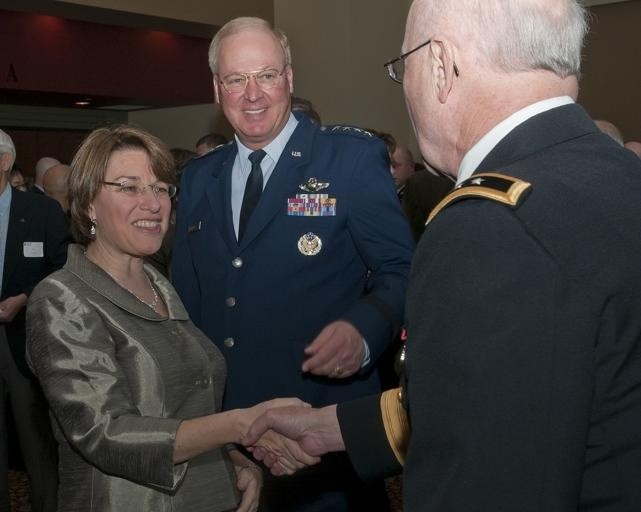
top-left (237, 148), bottom-right (268, 245)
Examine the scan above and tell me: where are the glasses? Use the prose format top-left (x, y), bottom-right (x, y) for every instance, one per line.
top-left (383, 38), bottom-right (460, 87)
top-left (216, 63), bottom-right (289, 94)
top-left (102, 178), bottom-right (178, 200)
